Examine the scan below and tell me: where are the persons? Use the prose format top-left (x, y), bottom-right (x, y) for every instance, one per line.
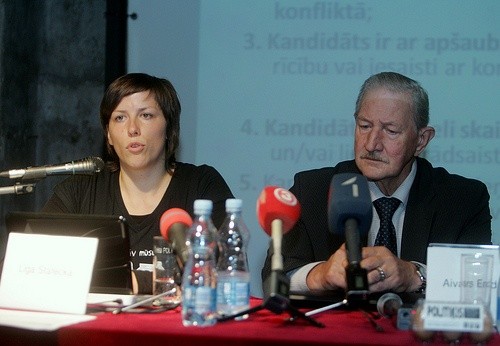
top-left (40, 73), bottom-right (246, 296)
top-left (261, 72), bottom-right (492, 299)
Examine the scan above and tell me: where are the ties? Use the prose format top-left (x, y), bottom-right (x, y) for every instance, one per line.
top-left (370, 196), bottom-right (402, 258)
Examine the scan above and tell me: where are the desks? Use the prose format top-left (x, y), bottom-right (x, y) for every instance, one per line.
top-left (0, 293), bottom-right (500, 346)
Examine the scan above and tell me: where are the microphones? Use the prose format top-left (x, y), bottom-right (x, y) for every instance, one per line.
top-left (0, 156), bottom-right (105, 181)
top-left (377, 293), bottom-right (414, 331)
top-left (327, 171), bottom-right (373, 291)
top-left (256, 185), bottom-right (301, 271)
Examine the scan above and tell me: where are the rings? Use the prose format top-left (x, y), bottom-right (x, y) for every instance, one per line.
top-left (377, 267), bottom-right (385, 280)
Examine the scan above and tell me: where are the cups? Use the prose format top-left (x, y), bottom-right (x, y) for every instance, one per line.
top-left (151, 235), bottom-right (180, 313)
top-left (459, 251), bottom-right (492, 316)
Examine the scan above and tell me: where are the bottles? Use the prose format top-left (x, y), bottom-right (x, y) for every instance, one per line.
top-left (180, 199), bottom-right (221, 327)
top-left (217, 198), bottom-right (251, 322)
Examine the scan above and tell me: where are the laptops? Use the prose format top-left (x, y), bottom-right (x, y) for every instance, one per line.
top-left (5, 211), bottom-right (135, 296)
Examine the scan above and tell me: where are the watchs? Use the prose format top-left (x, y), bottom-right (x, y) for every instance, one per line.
top-left (410, 261), bottom-right (426, 294)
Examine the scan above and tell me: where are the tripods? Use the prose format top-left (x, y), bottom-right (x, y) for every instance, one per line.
top-left (285, 294), bottom-right (387, 334)
top-left (217, 271), bottom-right (326, 329)
